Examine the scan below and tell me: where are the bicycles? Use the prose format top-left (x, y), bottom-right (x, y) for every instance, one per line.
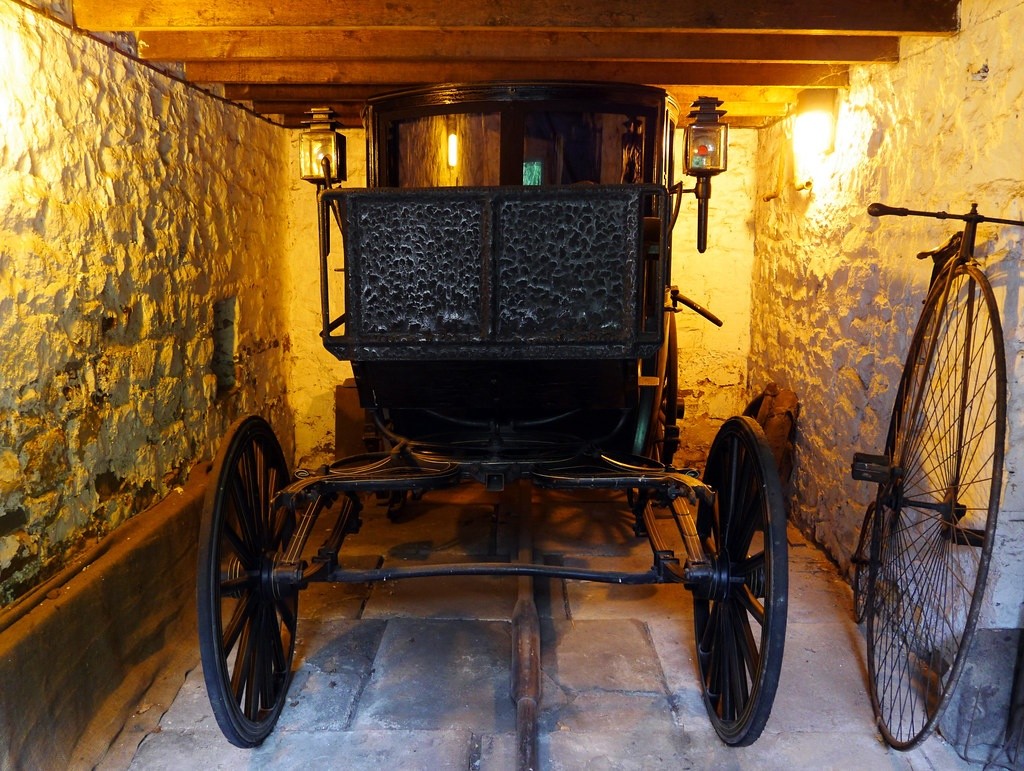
top-left (850, 203), bottom-right (1024, 752)
